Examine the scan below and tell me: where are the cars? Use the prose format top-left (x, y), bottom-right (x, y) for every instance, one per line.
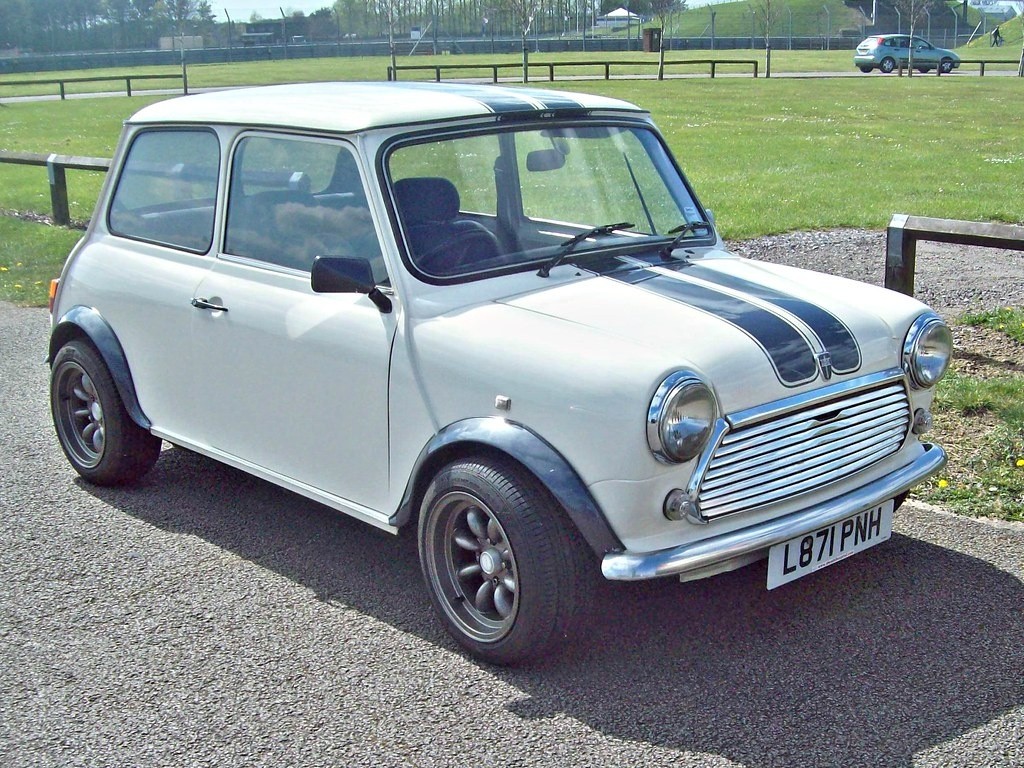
top-left (853, 33), bottom-right (960, 73)
top-left (42, 81), bottom-right (955, 666)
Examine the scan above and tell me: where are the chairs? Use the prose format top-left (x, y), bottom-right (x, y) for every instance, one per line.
top-left (365, 175), bottom-right (510, 277)
top-left (245, 190), bottom-right (325, 249)
